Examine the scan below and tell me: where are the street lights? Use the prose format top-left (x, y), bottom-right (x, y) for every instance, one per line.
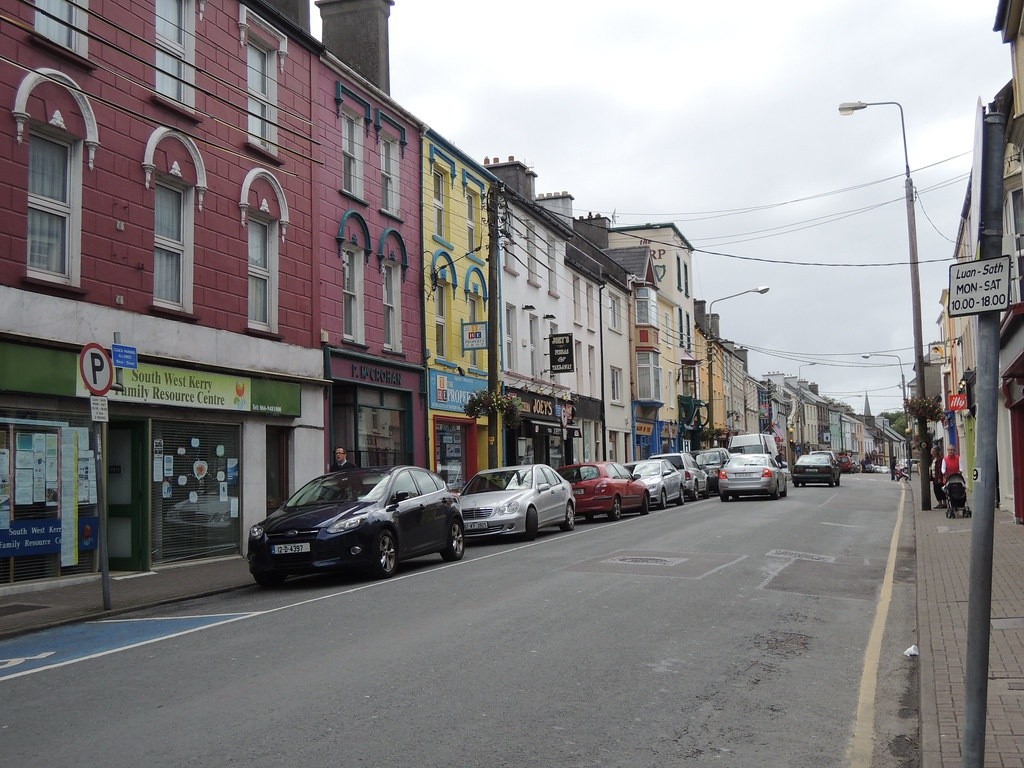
top-left (838, 99), bottom-right (934, 513)
top-left (707, 285), bottom-right (770, 445)
top-left (798, 362), bottom-right (817, 452)
top-left (862, 354), bottom-right (912, 481)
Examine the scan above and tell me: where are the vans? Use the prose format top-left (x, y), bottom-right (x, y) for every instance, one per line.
top-left (727, 434), bottom-right (788, 473)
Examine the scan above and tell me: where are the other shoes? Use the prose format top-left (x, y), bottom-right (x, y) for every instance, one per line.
top-left (933, 505), bottom-right (947, 509)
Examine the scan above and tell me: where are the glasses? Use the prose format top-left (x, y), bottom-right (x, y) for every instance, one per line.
top-left (335, 452), bottom-right (345, 455)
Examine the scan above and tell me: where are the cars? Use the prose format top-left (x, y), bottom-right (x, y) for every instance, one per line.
top-left (164, 476), bottom-right (242, 545)
top-left (454, 465), bottom-right (576, 540)
top-left (807, 449), bottom-right (923, 475)
top-left (248, 464), bottom-right (466, 590)
top-left (793, 455), bottom-right (840, 486)
top-left (625, 459), bottom-right (684, 509)
top-left (557, 463), bottom-right (651, 522)
top-left (719, 453), bottom-right (787, 502)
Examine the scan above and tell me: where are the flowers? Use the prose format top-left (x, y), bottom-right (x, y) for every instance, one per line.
top-left (903, 396), bottom-right (946, 423)
top-left (460, 390), bottom-right (524, 431)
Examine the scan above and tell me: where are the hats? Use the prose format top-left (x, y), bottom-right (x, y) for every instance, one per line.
top-left (947, 444), bottom-right (955, 449)
top-left (933, 445), bottom-right (941, 451)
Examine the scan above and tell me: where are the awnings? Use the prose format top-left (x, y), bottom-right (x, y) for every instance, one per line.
top-left (521, 419), bottom-right (582, 438)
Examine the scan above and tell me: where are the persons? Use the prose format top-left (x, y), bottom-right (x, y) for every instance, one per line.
top-left (941, 445), bottom-right (964, 481)
top-left (330, 447), bottom-right (359, 472)
top-left (890, 456), bottom-right (897, 480)
top-left (932, 446), bottom-right (948, 509)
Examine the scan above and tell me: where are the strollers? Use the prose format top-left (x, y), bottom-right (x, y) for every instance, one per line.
top-left (941, 472), bottom-right (972, 519)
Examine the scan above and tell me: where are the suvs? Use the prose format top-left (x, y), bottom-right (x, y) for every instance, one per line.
top-left (691, 448), bottom-right (733, 493)
top-left (648, 452), bottom-right (711, 502)
